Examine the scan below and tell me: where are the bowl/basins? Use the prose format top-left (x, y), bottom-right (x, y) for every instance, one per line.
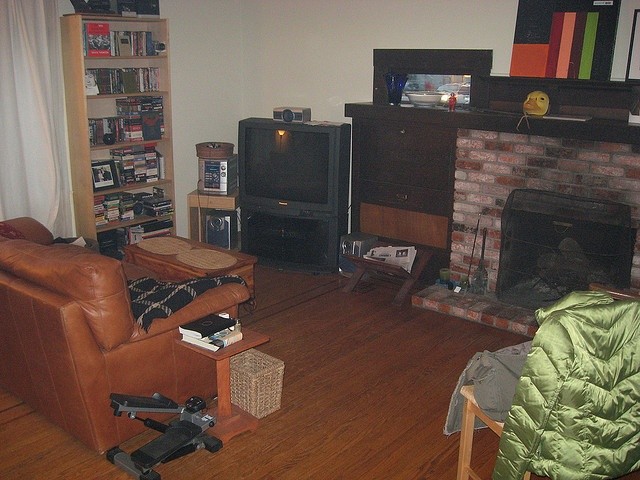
top-left (406, 93), bottom-right (443, 108)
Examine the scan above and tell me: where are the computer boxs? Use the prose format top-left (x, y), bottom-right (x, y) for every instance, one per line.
top-left (198, 154), bottom-right (237, 195)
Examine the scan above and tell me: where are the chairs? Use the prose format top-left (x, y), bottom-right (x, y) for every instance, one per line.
top-left (456, 384), bottom-right (531, 480)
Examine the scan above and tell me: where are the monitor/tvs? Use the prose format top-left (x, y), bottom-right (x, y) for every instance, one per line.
top-left (238, 116), bottom-right (351, 218)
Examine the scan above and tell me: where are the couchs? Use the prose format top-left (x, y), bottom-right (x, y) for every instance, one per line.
top-left (0, 217), bottom-right (251, 455)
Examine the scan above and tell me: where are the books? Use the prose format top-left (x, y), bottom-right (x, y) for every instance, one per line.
top-left (111, 146), bottom-right (164, 186)
top-left (85, 68), bottom-right (160, 94)
top-left (131, 221), bottom-right (173, 244)
top-left (181, 328), bottom-right (243, 353)
top-left (112, 31), bottom-right (153, 57)
top-left (94, 191), bottom-right (135, 227)
top-left (178, 313), bottom-right (236, 339)
top-left (88, 117), bottom-right (124, 147)
top-left (116, 97), bottom-right (165, 142)
top-left (133, 192), bottom-right (175, 218)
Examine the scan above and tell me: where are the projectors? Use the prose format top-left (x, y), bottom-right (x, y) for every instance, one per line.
top-left (273, 107), bottom-right (312, 123)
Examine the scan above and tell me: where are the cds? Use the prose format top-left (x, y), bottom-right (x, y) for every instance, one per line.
top-left (103, 134), bottom-right (115, 145)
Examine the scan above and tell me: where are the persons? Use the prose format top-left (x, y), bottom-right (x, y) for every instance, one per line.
top-left (82, 22), bottom-right (111, 58)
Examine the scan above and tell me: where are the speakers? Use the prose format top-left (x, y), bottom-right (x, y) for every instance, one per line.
top-left (205, 210), bottom-right (237, 251)
top-left (338, 231), bottom-right (378, 279)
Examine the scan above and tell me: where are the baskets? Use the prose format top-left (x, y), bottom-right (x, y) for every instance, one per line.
top-left (195, 141), bottom-right (234, 159)
top-left (229, 348), bottom-right (285, 420)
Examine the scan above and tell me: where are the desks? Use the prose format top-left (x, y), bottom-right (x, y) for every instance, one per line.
top-left (174, 328), bottom-right (271, 444)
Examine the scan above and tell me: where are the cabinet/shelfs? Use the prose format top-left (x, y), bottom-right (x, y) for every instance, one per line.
top-left (239, 203), bottom-right (337, 271)
top-left (344, 100), bottom-right (457, 250)
top-left (188, 190), bottom-right (242, 252)
top-left (61, 14), bottom-right (176, 259)
